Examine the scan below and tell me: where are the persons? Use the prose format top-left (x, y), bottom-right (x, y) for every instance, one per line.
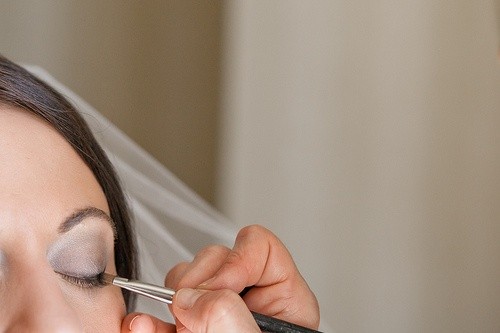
top-left (120, 224), bottom-right (320, 333)
top-left (0, 52), bottom-right (138, 333)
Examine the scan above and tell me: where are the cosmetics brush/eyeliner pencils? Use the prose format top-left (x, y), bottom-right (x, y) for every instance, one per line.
top-left (97, 272), bottom-right (326, 333)
